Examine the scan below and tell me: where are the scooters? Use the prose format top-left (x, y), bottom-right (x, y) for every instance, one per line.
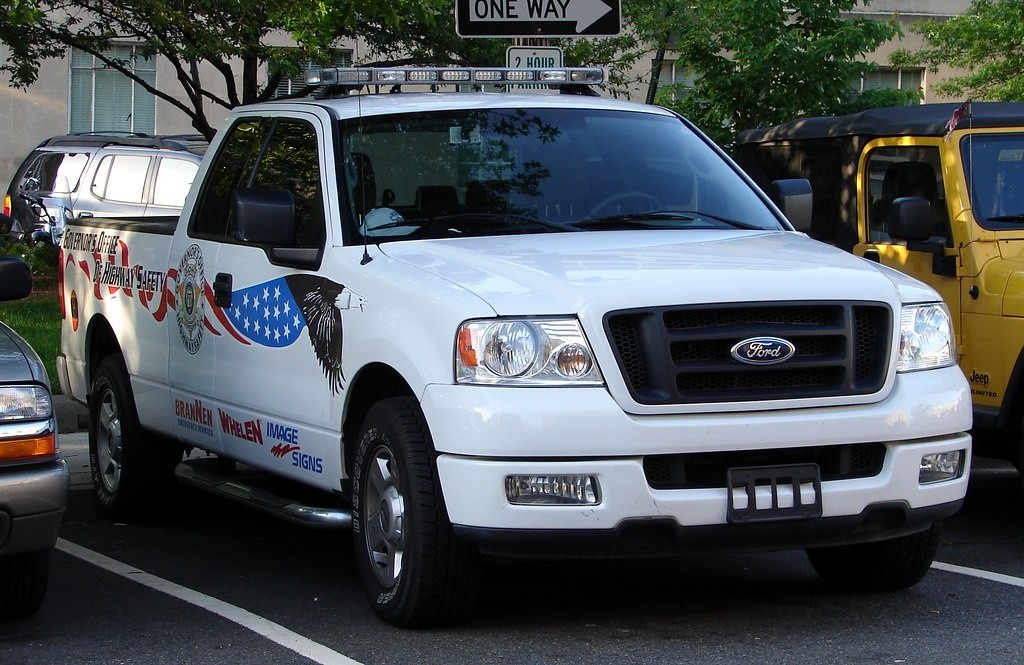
top-left (18, 194), bottom-right (93, 255)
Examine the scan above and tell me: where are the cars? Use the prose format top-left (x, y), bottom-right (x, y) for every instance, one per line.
top-left (0, 254), bottom-right (71, 622)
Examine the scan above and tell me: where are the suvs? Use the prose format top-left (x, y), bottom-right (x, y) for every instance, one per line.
top-left (3, 130), bottom-right (208, 252)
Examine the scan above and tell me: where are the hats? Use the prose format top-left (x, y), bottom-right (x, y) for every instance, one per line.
top-left (358, 207), bottom-right (421, 237)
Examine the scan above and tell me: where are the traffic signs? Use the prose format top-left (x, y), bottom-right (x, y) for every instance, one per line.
top-left (453, 0), bottom-right (624, 40)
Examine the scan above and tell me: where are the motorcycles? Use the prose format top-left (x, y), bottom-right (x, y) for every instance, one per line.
top-left (727, 101), bottom-right (1024, 479)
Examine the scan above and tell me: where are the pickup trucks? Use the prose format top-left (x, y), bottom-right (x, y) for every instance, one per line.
top-left (54, 61), bottom-right (977, 631)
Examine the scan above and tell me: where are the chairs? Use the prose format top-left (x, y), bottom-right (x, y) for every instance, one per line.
top-left (515, 137), bottom-right (576, 217)
top-left (345, 152), bottom-right (376, 224)
top-left (881, 161), bottom-right (939, 236)
